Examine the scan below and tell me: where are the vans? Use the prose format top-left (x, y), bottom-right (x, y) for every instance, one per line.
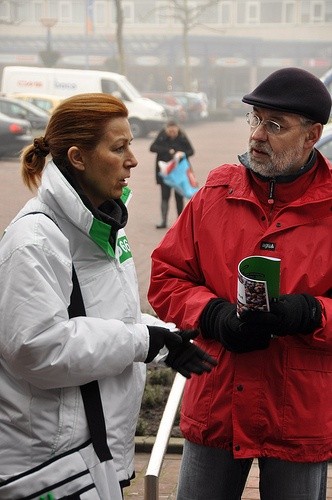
top-left (1, 67), bottom-right (167, 138)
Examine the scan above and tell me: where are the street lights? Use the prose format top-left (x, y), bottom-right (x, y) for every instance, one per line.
top-left (40, 18), bottom-right (60, 67)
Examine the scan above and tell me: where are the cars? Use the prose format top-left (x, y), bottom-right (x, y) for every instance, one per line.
top-left (1, 92), bottom-right (209, 158)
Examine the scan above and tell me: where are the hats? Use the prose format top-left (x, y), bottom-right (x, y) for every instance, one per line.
top-left (242, 66), bottom-right (332, 125)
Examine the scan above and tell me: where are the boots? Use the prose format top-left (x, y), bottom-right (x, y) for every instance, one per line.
top-left (176, 199), bottom-right (183, 217)
top-left (156, 196), bottom-right (169, 229)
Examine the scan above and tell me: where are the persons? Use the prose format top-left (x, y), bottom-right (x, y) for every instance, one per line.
top-left (150, 122), bottom-right (193, 229)
top-left (146, 67), bottom-right (332, 500)
top-left (0, 93), bottom-right (217, 500)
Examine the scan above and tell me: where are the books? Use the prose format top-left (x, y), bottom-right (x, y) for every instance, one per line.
top-left (237, 255), bottom-right (281, 319)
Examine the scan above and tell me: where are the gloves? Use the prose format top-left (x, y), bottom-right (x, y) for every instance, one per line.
top-left (143, 326), bottom-right (218, 378)
top-left (199, 296), bottom-right (273, 355)
top-left (266, 293), bottom-right (323, 339)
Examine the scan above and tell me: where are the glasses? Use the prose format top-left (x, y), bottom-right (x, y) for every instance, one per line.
top-left (244, 112), bottom-right (292, 135)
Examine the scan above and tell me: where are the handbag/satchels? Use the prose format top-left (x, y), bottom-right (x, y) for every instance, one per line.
top-left (155, 152), bottom-right (199, 199)
